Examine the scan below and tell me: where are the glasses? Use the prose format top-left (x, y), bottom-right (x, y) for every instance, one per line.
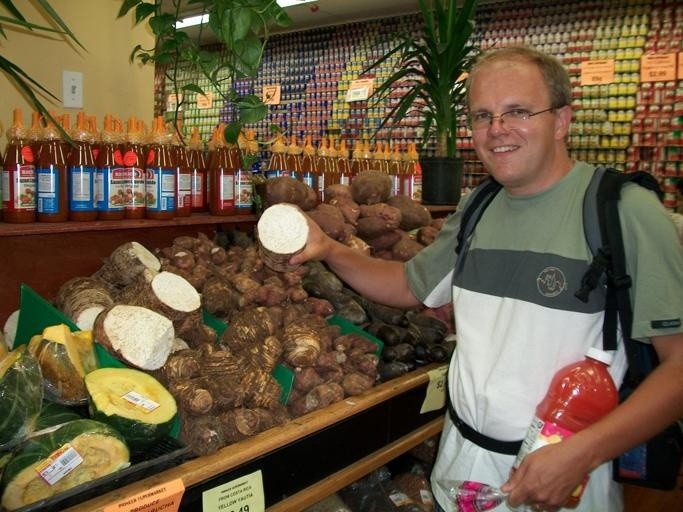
top-left (461, 103), bottom-right (570, 131)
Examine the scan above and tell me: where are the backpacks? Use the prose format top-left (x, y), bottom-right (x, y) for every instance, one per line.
top-left (444, 167), bottom-right (680, 491)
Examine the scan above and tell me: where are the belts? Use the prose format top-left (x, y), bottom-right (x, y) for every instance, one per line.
top-left (443, 370), bottom-right (524, 461)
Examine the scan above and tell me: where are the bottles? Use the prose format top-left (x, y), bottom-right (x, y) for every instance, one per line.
top-left (506, 347), bottom-right (619, 508)
top-left (0, 104), bottom-right (424, 225)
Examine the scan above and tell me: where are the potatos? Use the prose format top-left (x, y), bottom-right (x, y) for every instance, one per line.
top-left (262, 169), bottom-right (452, 325)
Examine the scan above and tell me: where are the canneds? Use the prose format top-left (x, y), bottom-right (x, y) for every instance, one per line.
top-left (420, 149), bottom-right (682, 214)
top-left (154, 1), bottom-right (682, 149)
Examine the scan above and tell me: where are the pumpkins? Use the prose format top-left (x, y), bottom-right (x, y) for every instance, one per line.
top-left (0, 323), bottom-right (177, 510)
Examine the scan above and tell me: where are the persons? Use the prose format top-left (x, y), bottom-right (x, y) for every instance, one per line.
top-left (281, 46), bottom-right (682, 511)
